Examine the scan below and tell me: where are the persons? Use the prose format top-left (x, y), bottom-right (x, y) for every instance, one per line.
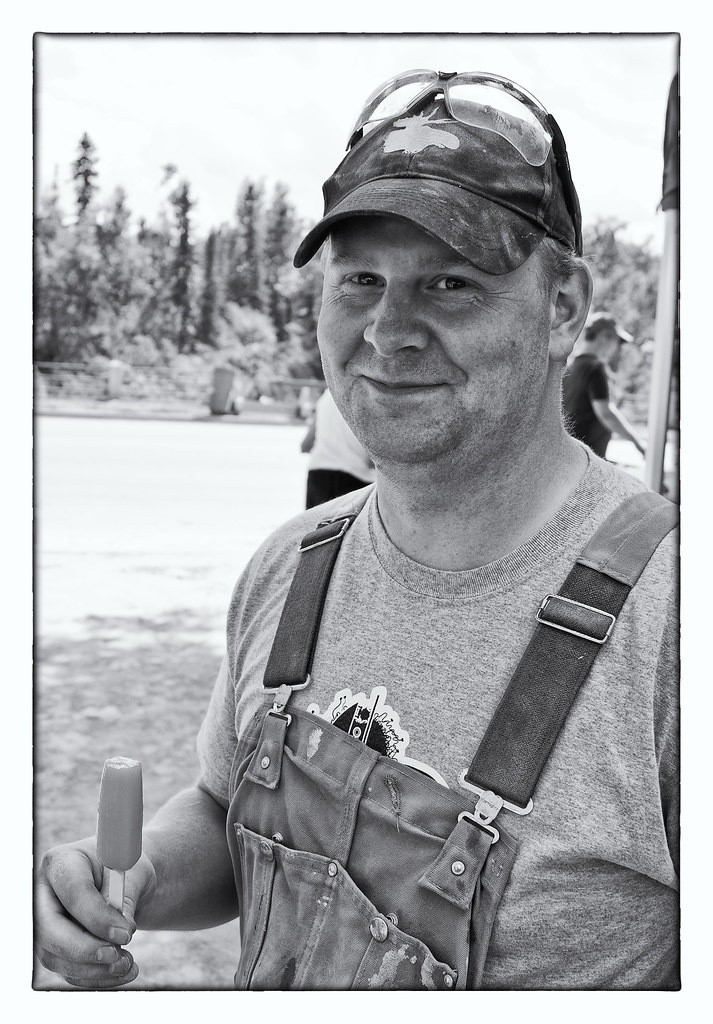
top-left (34, 70), bottom-right (681, 990)
top-left (562, 312), bottom-right (646, 462)
top-left (301, 387), bottom-right (377, 510)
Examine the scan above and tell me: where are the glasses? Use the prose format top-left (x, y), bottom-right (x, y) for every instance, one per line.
top-left (346, 69), bottom-right (585, 257)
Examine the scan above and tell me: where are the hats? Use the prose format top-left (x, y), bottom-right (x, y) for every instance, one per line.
top-left (294, 98), bottom-right (577, 275)
